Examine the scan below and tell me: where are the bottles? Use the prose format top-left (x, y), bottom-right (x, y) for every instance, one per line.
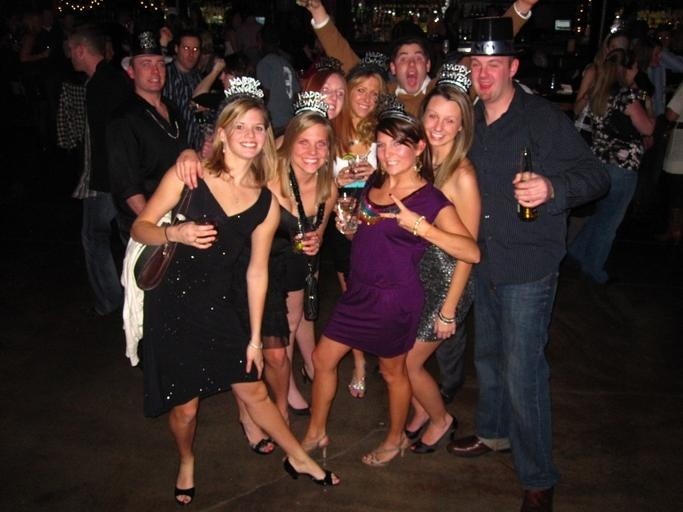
top-left (349, 2), bottom-right (439, 40)
top-left (262, 15), bottom-right (273, 40)
top-left (538, 73), bottom-right (560, 94)
top-left (516, 145), bottom-right (538, 224)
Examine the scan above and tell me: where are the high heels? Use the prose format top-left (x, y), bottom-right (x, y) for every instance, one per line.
top-left (238, 360), bottom-right (365, 487)
top-left (172, 481), bottom-right (195, 503)
top-left (361, 413), bottom-right (457, 467)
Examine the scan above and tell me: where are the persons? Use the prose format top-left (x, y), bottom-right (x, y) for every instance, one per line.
top-left (1, 1), bottom-right (683, 510)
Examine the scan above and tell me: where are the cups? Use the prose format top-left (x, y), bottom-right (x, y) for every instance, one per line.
top-left (336, 197), bottom-right (359, 236)
top-left (201, 220), bottom-right (224, 246)
top-left (289, 218), bottom-right (314, 256)
top-left (348, 154), bottom-right (367, 180)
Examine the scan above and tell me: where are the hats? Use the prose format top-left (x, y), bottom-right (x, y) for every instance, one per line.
top-left (455, 15), bottom-right (525, 58)
top-left (120, 32), bottom-right (172, 72)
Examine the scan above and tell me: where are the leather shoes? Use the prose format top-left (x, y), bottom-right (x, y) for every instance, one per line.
top-left (519, 485), bottom-right (555, 512)
top-left (446, 432), bottom-right (512, 458)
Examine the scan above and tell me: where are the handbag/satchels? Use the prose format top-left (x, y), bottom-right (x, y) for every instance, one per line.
top-left (132, 222), bottom-right (178, 292)
